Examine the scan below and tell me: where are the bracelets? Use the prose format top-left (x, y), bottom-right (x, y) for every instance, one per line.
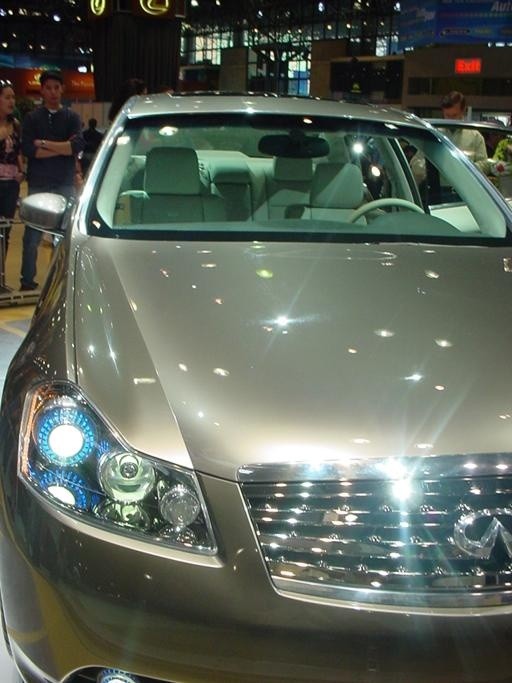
top-left (41, 140), bottom-right (46, 147)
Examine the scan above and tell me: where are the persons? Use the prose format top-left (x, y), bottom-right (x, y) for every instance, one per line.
top-left (0, 78), bottom-right (25, 302)
top-left (409, 91), bottom-right (490, 205)
top-left (19, 68), bottom-right (85, 291)
top-left (78, 118), bottom-right (103, 173)
top-left (108, 77), bottom-right (147, 121)
top-left (468, 133), bottom-right (512, 198)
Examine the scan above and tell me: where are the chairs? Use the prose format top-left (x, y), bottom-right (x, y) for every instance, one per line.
top-left (129, 147), bottom-right (368, 228)
